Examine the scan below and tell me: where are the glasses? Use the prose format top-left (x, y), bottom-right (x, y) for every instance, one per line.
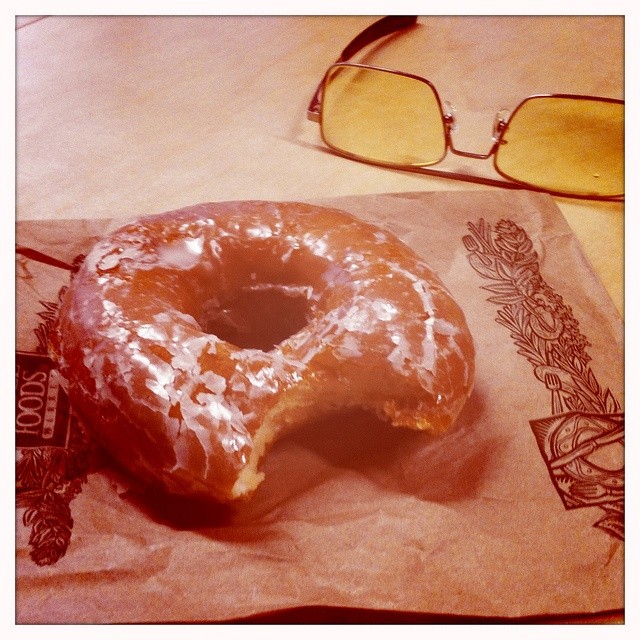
top-left (308, 15), bottom-right (624, 199)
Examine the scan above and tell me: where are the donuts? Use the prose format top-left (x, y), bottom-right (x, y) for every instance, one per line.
top-left (60, 199), bottom-right (475, 504)
